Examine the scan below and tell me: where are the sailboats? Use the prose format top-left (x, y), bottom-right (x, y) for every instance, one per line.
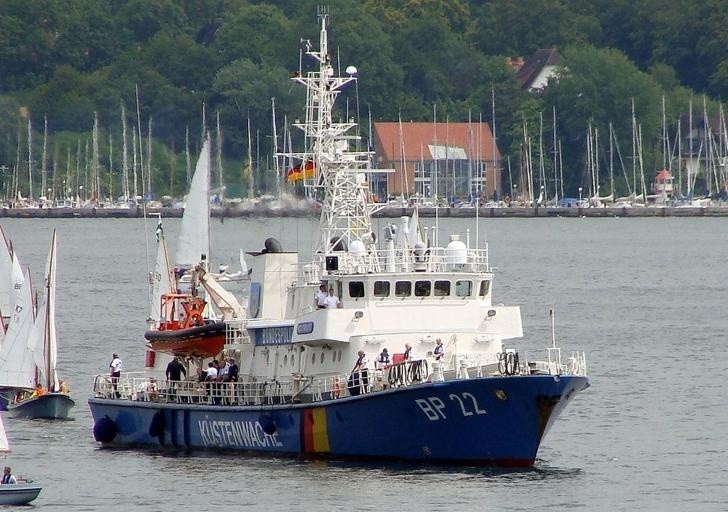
top-left (0, 220), bottom-right (75, 505)
top-left (145, 209), bottom-right (230, 355)
top-left (151, 131), bottom-right (253, 289)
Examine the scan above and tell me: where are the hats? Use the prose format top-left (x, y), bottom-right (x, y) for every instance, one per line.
top-left (4, 467), bottom-right (12, 471)
top-left (113, 353), bottom-right (120, 357)
top-left (359, 351), bottom-right (366, 354)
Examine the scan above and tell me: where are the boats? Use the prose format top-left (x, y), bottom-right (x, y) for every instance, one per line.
top-left (87, 6), bottom-right (590, 473)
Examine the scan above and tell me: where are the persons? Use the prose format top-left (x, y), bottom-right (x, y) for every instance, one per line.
top-left (0, 466), bottom-right (17, 484)
top-left (404, 342), bottom-right (415, 361)
top-left (109, 351), bottom-right (122, 399)
top-left (196, 357), bottom-right (239, 405)
top-left (374, 348), bottom-right (393, 368)
top-left (314, 284), bottom-right (341, 309)
top-left (12, 391), bottom-right (24, 404)
top-left (432, 337), bottom-right (446, 361)
top-left (34, 384), bottom-right (45, 396)
top-left (166, 356), bottom-right (187, 402)
top-left (58, 378), bottom-right (69, 393)
top-left (351, 350), bottom-right (368, 372)
